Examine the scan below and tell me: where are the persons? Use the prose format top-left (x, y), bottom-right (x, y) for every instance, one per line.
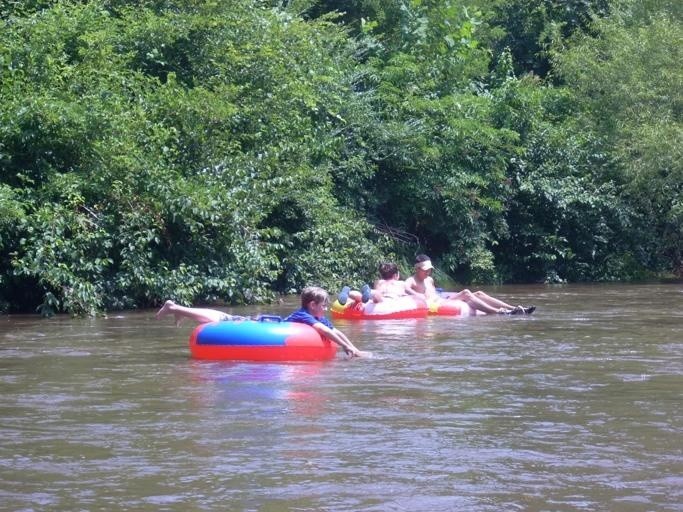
top-left (337, 261), bottom-right (427, 306)
top-left (403, 253), bottom-right (535, 316)
top-left (155, 286), bottom-right (359, 360)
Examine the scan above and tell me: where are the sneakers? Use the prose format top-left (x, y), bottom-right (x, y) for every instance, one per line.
top-left (361, 285), bottom-right (371, 303)
top-left (501, 306), bottom-right (525, 315)
top-left (524, 306), bottom-right (536, 314)
top-left (339, 286), bottom-right (350, 305)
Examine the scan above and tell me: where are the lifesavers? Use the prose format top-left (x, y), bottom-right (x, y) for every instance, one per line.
top-left (189, 315), bottom-right (338, 361)
top-left (331, 298), bottom-right (427, 320)
top-left (429, 289), bottom-right (487, 316)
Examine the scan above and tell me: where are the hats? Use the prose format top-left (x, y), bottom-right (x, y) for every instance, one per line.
top-left (414, 259), bottom-right (435, 271)
